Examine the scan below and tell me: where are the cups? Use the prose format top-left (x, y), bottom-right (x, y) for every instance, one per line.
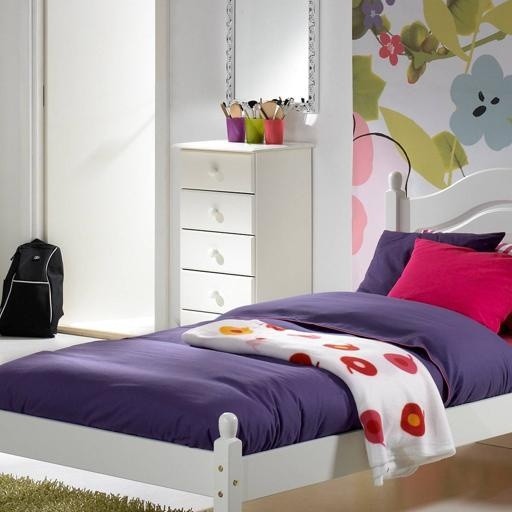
top-left (226, 117), bottom-right (245, 142)
top-left (264, 119), bottom-right (283, 145)
top-left (245, 118), bottom-right (264, 143)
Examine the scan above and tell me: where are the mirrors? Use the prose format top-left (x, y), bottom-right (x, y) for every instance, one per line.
top-left (225, 0), bottom-right (320, 114)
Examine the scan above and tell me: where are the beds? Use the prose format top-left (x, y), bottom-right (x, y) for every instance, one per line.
top-left (0, 167), bottom-right (512, 512)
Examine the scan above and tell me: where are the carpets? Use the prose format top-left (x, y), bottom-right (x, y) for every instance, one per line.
top-left (0, 472), bottom-right (197, 512)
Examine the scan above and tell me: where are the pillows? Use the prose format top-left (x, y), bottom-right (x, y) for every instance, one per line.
top-left (386, 237), bottom-right (512, 335)
top-left (409, 228), bottom-right (512, 330)
top-left (355, 229), bottom-right (505, 296)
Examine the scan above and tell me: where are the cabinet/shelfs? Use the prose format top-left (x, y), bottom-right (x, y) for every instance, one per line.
top-left (169, 138), bottom-right (315, 327)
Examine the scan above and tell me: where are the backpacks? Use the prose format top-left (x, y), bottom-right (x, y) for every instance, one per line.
top-left (0, 241), bottom-right (63, 338)
